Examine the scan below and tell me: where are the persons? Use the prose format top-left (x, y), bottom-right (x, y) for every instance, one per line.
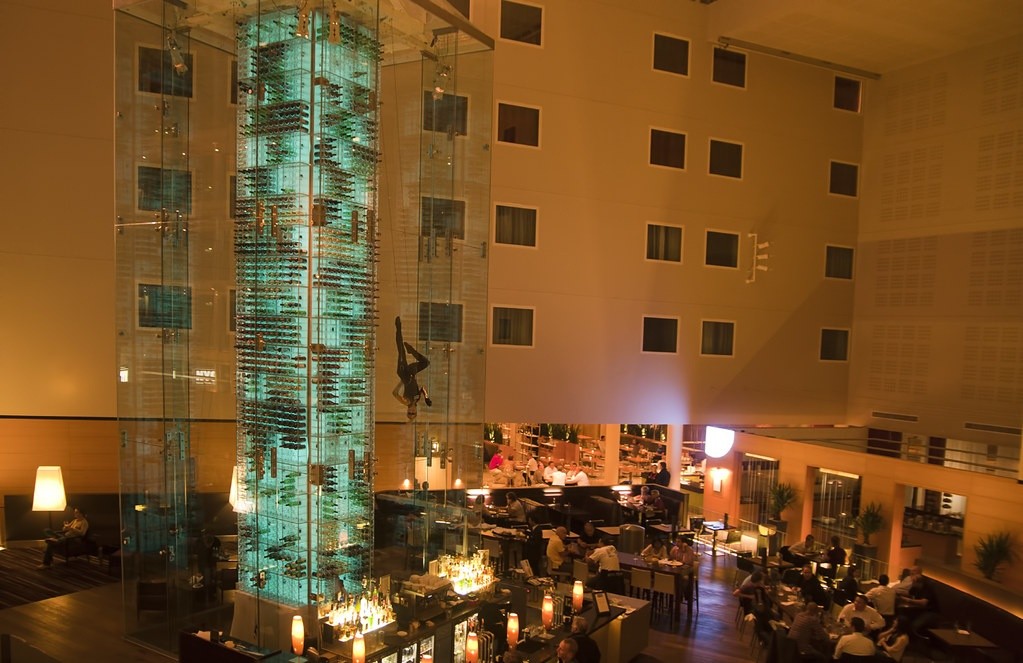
top-left (391, 316), bottom-right (433, 420)
top-left (31, 506), bottom-right (89, 570)
top-left (192, 528), bottom-right (221, 599)
top-left (416, 436), bottom-right (936, 663)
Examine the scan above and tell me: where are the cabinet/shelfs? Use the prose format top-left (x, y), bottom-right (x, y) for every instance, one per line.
top-left (515, 422), bottom-right (542, 460)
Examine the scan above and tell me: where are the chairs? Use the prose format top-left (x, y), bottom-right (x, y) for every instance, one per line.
top-left (630, 566), bottom-right (651, 601)
top-left (652, 571), bottom-right (679, 631)
top-left (483, 538), bottom-right (504, 575)
top-left (54, 523), bottom-right (92, 568)
top-left (735, 596), bottom-right (767, 662)
top-left (717, 530), bottom-right (745, 562)
top-left (402, 513), bottom-right (425, 570)
top-left (572, 560), bottom-right (594, 584)
top-left (678, 560), bottom-right (701, 615)
top-left (689, 516), bottom-right (712, 546)
top-left (546, 554), bottom-right (572, 580)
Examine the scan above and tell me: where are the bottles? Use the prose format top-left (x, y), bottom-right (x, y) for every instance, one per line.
top-left (437, 552), bottom-right (496, 592)
top-left (343, 618), bottom-right (347, 635)
top-left (350, 613), bottom-right (354, 628)
top-left (245, 472), bottom-right (301, 506)
top-left (316, 459), bottom-right (373, 549)
top-left (264, 535), bottom-right (306, 579)
top-left (234, 7), bottom-right (385, 471)
top-left (356, 595), bottom-right (360, 611)
top-left (373, 586), bottom-right (378, 607)
top-left (362, 605), bottom-right (393, 632)
top-left (360, 593), bottom-right (367, 611)
top-left (329, 604), bottom-right (334, 624)
top-left (250, 570), bottom-right (271, 589)
top-left (337, 623), bottom-right (341, 639)
top-left (357, 619), bottom-right (363, 634)
top-left (347, 593), bottom-right (353, 606)
top-left (346, 622), bottom-right (351, 638)
top-left (356, 612), bottom-right (361, 622)
top-left (368, 597), bottom-right (373, 607)
top-left (241, 524), bottom-right (270, 552)
top-left (386, 590), bottom-right (390, 606)
top-left (378, 585), bottom-right (383, 606)
top-left (355, 615), bottom-right (359, 627)
top-left (312, 544), bottom-right (370, 578)
top-left (340, 593), bottom-right (344, 603)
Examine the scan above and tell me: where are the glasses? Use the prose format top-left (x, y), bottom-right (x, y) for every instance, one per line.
top-left (408, 412), bottom-right (414, 415)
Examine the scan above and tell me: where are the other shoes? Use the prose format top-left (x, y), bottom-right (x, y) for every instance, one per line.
top-left (36, 564), bottom-right (53, 570)
top-left (45, 538), bottom-right (57, 544)
top-left (395, 316), bottom-right (400, 328)
top-left (404, 342), bottom-right (412, 354)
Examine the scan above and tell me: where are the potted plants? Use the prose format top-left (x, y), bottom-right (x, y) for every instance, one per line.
top-left (767, 480), bottom-right (799, 539)
top-left (851, 498), bottom-right (889, 564)
top-left (967, 526), bottom-right (1023, 588)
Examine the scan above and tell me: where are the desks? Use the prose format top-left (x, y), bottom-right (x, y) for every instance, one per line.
top-left (618, 551), bottom-right (695, 630)
top-left (486, 433), bottom-right (703, 484)
top-left (702, 519), bottom-right (736, 557)
top-left (487, 506), bottom-right (519, 520)
top-left (764, 581), bottom-right (855, 644)
top-left (626, 499), bottom-right (663, 534)
top-left (468, 524), bottom-right (527, 573)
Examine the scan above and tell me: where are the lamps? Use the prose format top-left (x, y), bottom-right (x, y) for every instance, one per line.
top-left (573, 578), bottom-right (583, 624)
top-left (288, 615), bottom-right (309, 663)
top-left (420, 654), bottom-right (433, 663)
top-left (506, 612), bottom-right (520, 652)
top-left (351, 632), bottom-right (366, 663)
top-left (466, 631), bottom-right (480, 663)
top-left (30, 464), bottom-right (69, 530)
top-left (538, 593), bottom-right (556, 641)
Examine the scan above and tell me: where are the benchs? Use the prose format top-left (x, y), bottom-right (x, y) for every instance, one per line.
top-left (519, 497), bottom-right (568, 538)
top-left (651, 495), bottom-right (686, 526)
top-left (588, 494), bottom-right (633, 538)
top-left (549, 503), bottom-right (591, 531)
